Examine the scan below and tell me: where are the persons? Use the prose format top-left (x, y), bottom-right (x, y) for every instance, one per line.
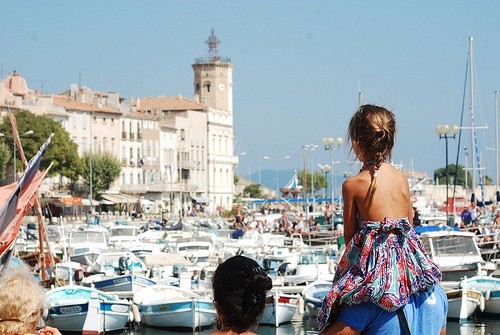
top-left (319, 282), bottom-right (448, 335)
top-left (454, 191), bottom-right (500, 259)
top-left (411, 206), bottom-right (421, 227)
top-left (228, 199), bottom-right (343, 243)
top-left (86, 210), bottom-right (91, 223)
top-left (342, 104), bottom-right (436, 297)
top-left (0, 266), bottom-right (63, 335)
top-left (208, 255), bottom-right (273, 335)
top-left (186, 201), bottom-right (205, 218)
top-left (161, 209), bottom-right (168, 227)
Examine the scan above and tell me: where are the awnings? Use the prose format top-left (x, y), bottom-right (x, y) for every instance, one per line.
top-left (98, 192), bottom-right (142, 204)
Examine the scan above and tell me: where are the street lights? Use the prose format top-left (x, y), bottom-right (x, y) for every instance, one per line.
top-left (322, 137), bottom-right (344, 241)
top-left (318, 163), bottom-right (332, 227)
top-left (304, 144), bottom-right (318, 221)
top-left (434, 124), bottom-right (459, 226)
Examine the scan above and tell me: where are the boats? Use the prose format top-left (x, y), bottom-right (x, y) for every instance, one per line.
top-left (14, 218), bottom-right (500, 335)
